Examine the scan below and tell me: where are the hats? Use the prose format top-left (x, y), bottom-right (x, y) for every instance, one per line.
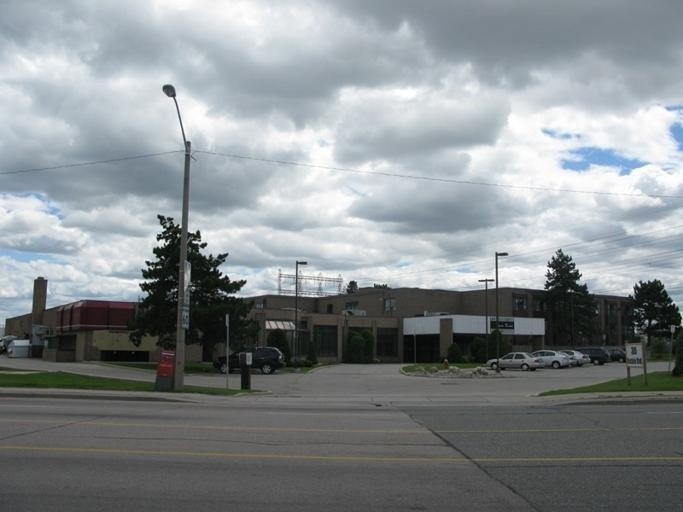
top-left (610, 349), bottom-right (626, 363)
top-left (485, 347), bottom-right (609, 372)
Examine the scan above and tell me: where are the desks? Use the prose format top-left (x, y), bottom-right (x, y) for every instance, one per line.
top-left (294, 261), bottom-right (307, 357)
top-left (478, 252), bottom-right (509, 359)
top-left (162, 84), bottom-right (191, 389)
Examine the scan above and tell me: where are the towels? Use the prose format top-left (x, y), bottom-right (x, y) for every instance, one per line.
top-left (153, 350), bottom-right (176, 392)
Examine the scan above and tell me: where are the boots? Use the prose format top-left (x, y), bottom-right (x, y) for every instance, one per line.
top-left (214, 347), bottom-right (286, 375)
